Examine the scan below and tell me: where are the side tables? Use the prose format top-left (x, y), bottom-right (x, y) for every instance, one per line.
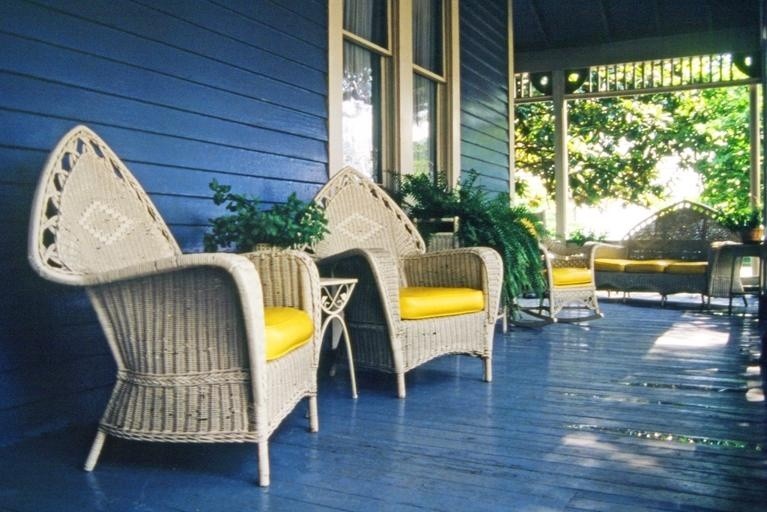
top-left (707, 241), bottom-right (767, 316)
top-left (318, 277), bottom-right (359, 400)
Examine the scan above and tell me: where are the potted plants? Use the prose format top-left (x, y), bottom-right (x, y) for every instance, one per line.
top-left (714, 192), bottom-right (765, 244)
top-left (202, 177), bottom-right (331, 252)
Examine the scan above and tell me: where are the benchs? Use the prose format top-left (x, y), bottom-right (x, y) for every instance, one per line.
top-left (583, 199), bottom-right (741, 306)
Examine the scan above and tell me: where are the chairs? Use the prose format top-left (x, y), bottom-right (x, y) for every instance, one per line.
top-left (291, 165), bottom-right (504, 399)
top-left (535, 235), bottom-right (604, 323)
top-left (411, 215), bottom-right (507, 333)
top-left (26, 123), bottom-right (322, 486)
